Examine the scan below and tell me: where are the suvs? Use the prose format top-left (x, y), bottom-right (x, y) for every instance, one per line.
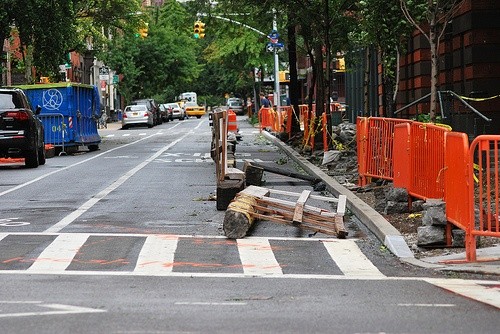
top-left (0, 86), bottom-right (47, 169)
top-left (129, 99), bottom-right (159, 126)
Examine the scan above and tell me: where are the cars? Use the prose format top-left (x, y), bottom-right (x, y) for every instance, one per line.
top-left (163, 103), bottom-right (174, 122)
top-left (150, 99), bottom-right (162, 125)
top-left (181, 102), bottom-right (206, 119)
top-left (226, 98), bottom-right (242, 107)
top-left (159, 103), bottom-right (168, 124)
top-left (229, 100), bottom-right (244, 114)
top-left (121, 105), bottom-right (154, 128)
top-left (167, 103), bottom-right (185, 120)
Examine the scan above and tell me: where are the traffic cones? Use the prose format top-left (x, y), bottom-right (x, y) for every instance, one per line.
top-left (227, 110), bottom-right (241, 139)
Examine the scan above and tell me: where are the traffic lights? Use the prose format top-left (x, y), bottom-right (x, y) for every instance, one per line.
top-left (133, 31), bottom-right (141, 39)
top-left (193, 21), bottom-right (202, 39)
top-left (201, 22), bottom-right (206, 39)
top-left (141, 20), bottom-right (149, 39)
